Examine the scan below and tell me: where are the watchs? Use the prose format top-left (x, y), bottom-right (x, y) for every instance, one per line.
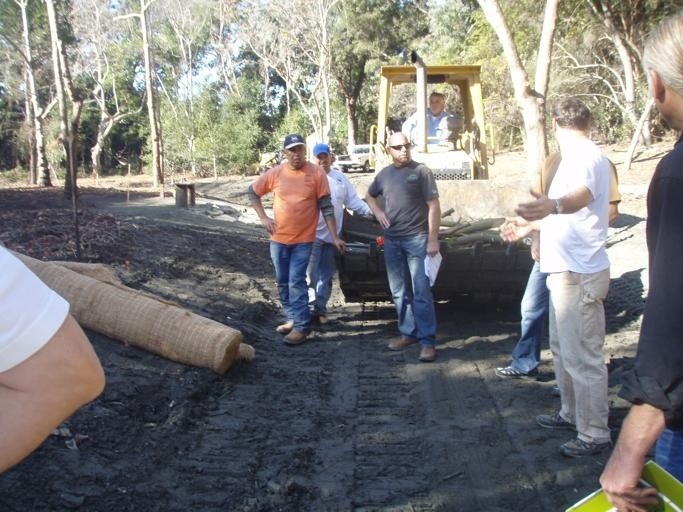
top-left (555, 199), bottom-right (563, 214)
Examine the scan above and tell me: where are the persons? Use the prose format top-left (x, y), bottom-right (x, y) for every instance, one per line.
top-left (599, 9), bottom-right (683, 511)
top-left (495, 151), bottom-right (620, 396)
top-left (402, 92), bottom-right (453, 145)
top-left (0, 243), bottom-right (107, 474)
top-left (515, 98), bottom-right (613, 459)
top-left (365, 132), bottom-right (440, 362)
top-left (248, 134), bottom-right (345, 345)
top-left (304, 144), bottom-right (372, 325)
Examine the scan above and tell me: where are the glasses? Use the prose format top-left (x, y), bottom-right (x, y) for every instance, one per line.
top-left (389, 143), bottom-right (411, 150)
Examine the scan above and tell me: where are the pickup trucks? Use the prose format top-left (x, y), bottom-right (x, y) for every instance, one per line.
top-left (336, 142), bottom-right (375, 173)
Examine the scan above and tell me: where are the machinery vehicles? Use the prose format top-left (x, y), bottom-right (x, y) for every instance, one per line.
top-left (336, 51), bottom-right (538, 308)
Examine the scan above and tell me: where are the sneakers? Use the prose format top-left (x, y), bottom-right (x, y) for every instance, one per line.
top-left (276, 322), bottom-right (294, 333)
top-left (319, 315), bottom-right (327, 324)
top-left (494, 366), bottom-right (538, 380)
top-left (536, 413), bottom-right (576, 431)
top-left (417, 342), bottom-right (436, 361)
top-left (283, 329), bottom-right (314, 344)
top-left (388, 338), bottom-right (418, 349)
top-left (560, 436), bottom-right (613, 457)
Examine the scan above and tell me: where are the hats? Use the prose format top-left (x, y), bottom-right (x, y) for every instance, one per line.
top-left (284, 134), bottom-right (304, 149)
top-left (313, 144), bottom-right (330, 157)
